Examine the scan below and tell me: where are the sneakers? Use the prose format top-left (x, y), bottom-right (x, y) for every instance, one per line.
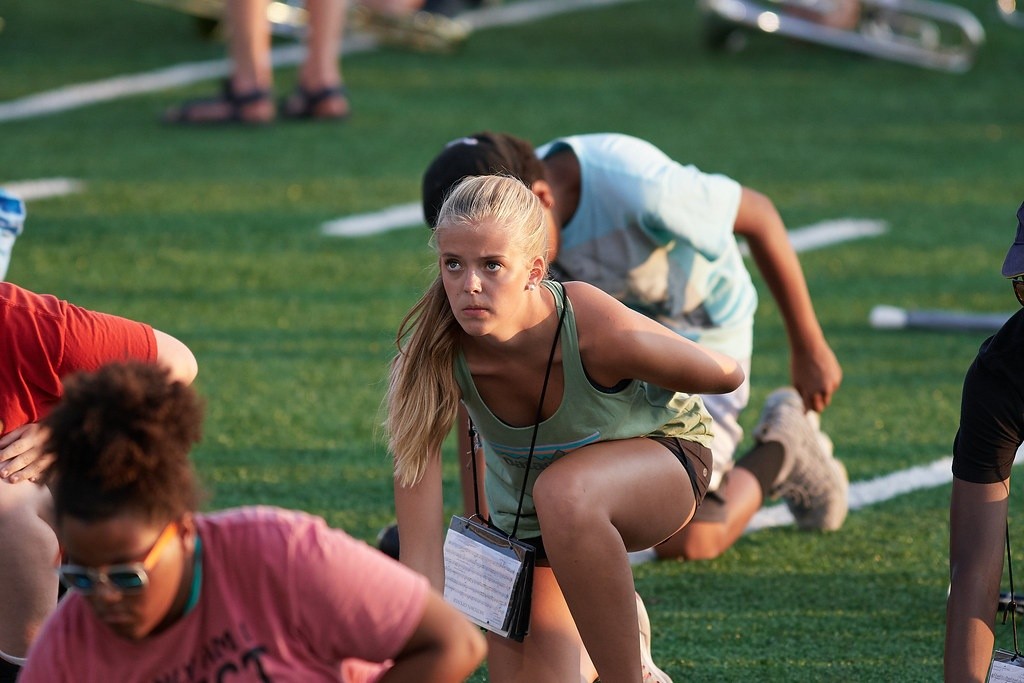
top-left (757, 388), bottom-right (852, 533)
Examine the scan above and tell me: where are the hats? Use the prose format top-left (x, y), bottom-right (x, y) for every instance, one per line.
top-left (1003, 203), bottom-right (1024, 278)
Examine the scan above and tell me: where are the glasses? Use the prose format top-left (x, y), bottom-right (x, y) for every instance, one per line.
top-left (1011, 275), bottom-right (1023, 306)
top-left (52, 522), bottom-right (175, 594)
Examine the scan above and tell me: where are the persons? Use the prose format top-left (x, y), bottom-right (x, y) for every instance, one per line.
top-left (394, 170), bottom-right (747, 682)
top-left (158, 0), bottom-right (353, 129)
top-left (420, 124), bottom-right (856, 569)
top-left (0, 272), bottom-right (199, 683)
top-left (7, 357), bottom-right (487, 682)
top-left (937, 202), bottom-right (1024, 683)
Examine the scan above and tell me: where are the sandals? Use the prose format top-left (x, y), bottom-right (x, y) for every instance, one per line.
top-left (158, 77), bottom-right (278, 127)
top-left (283, 80), bottom-right (355, 122)
